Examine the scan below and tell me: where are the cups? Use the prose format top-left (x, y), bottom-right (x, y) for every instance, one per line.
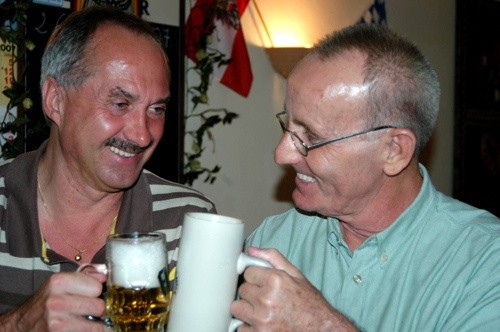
top-left (76, 231), bottom-right (168, 332)
top-left (167, 212), bottom-right (273, 332)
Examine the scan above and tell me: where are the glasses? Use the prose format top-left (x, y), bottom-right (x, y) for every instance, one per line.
top-left (276, 111), bottom-right (398, 156)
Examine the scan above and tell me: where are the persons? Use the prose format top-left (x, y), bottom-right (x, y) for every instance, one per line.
top-left (0, 5), bottom-right (220, 332)
top-left (229, 23), bottom-right (500, 332)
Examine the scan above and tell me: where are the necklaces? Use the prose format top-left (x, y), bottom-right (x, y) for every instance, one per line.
top-left (37, 180), bottom-right (112, 263)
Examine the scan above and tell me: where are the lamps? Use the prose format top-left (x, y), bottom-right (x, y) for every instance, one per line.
top-left (237, 0), bottom-right (377, 78)
